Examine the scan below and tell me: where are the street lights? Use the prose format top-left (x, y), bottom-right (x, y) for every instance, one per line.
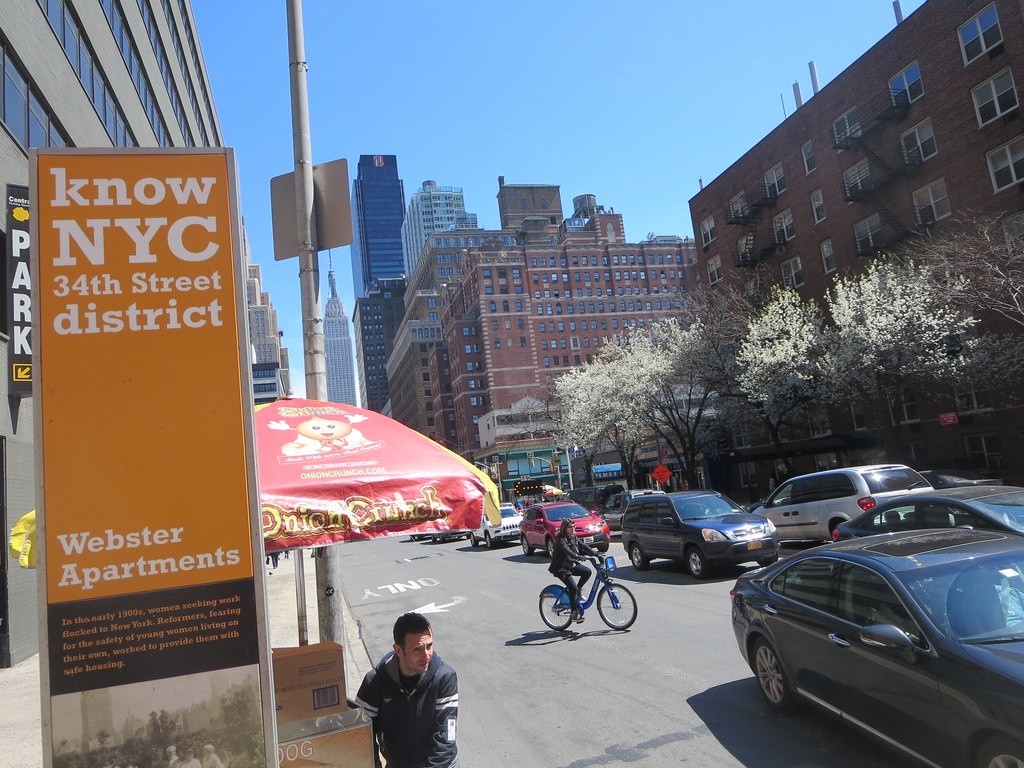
top-left (531, 456), bottom-right (561, 491)
top-left (475, 460), bottom-right (504, 502)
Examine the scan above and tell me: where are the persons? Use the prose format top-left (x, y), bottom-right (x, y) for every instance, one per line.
top-left (310, 548), bottom-right (315, 557)
top-left (264, 551), bottom-right (292, 569)
top-left (547, 518), bottom-right (603, 622)
top-left (355, 613), bottom-right (461, 768)
top-left (977, 569), bottom-right (1024, 631)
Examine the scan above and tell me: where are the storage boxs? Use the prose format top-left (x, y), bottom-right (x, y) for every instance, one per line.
top-left (271, 640), bottom-right (346, 726)
top-left (279, 703), bottom-right (373, 768)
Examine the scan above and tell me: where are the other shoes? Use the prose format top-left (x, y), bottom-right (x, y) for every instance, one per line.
top-left (570, 610), bottom-right (586, 622)
top-left (577, 588), bottom-right (587, 603)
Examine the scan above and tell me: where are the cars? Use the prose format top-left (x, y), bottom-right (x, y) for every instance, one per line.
top-left (750, 464), bottom-right (935, 543)
top-left (410, 531), bottom-right (472, 544)
top-left (832, 484), bottom-right (1024, 546)
top-left (915, 468), bottom-right (1005, 491)
top-left (729, 527), bottom-right (1024, 768)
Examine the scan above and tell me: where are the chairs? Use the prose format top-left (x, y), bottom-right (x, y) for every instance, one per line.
top-left (885, 511), bottom-right (900, 522)
top-left (799, 564), bottom-right (831, 580)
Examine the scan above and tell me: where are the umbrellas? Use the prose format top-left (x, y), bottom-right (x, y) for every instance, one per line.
top-left (8, 396), bottom-right (502, 644)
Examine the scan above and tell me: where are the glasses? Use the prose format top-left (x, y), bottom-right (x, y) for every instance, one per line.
top-left (567, 524), bottom-right (576, 528)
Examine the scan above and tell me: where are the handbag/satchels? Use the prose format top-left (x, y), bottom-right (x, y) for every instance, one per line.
top-left (549, 561), bottom-right (567, 580)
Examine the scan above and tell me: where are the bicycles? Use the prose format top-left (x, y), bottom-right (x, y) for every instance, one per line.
top-left (538, 552), bottom-right (638, 632)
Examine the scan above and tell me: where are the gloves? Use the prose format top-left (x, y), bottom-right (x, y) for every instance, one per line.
top-left (597, 552), bottom-right (603, 557)
top-left (577, 555), bottom-right (586, 562)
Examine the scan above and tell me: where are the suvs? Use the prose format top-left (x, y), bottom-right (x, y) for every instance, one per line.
top-left (469, 506), bottom-right (525, 549)
top-left (595, 489), bottom-right (666, 531)
top-left (561, 484), bottom-right (625, 514)
top-left (520, 500), bottom-right (611, 556)
top-left (622, 490), bottom-right (782, 580)
